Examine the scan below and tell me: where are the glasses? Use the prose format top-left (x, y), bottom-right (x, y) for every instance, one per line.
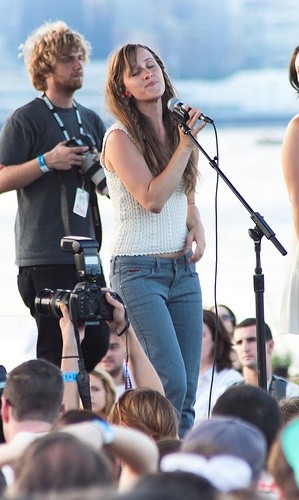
top-left (219, 314), bottom-right (234, 322)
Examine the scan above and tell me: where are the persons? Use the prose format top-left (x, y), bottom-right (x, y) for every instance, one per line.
top-left (99, 44), bottom-right (207, 441)
top-left (192, 309), bottom-right (244, 430)
top-left (0, 19), bottom-right (110, 376)
top-left (58, 289), bottom-right (179, 444)
top-left (233, 318), bottom-right (299, 402)
top-left (0, 359), bottom-right (299, 500)
top-left (209, 304), bottom-right (243, 376)
top-left (94, 328), bottom-right (136, 402)
top-left (279, 45), bottom-right (299, 337)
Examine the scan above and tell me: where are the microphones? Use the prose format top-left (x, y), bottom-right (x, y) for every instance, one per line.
top-left (167, 97), bottom-right (213, 124)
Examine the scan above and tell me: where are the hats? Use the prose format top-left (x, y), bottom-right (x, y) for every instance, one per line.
top-left (181, 415), bottom-right (267, 482)
top-left (280, 416), bottom-right (299, 490)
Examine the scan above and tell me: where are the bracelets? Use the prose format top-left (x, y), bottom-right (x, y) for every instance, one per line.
top-left (37, 155), bottom-right (52, 174)
top-left (61, 371), bottom-right (79, 382)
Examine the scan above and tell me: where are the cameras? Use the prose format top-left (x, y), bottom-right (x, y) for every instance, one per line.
top-left (67, 134), bottom-right (110, 199)
top-left (35, 235), bottom-right (116, 326)
